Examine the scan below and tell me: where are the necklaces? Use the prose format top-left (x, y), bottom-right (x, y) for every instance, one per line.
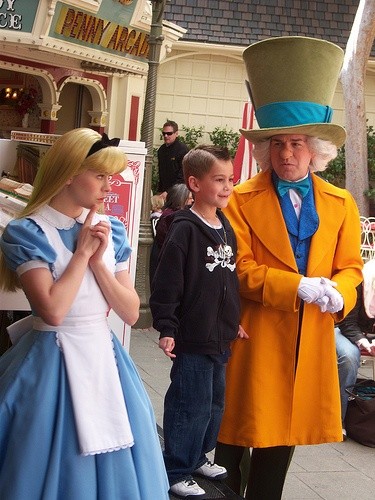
top-left (192, 203), bottom-right (227, 244)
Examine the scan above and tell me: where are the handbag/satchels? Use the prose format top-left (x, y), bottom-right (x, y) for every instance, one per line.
top-left (344, 379), bottom-right (375, 448)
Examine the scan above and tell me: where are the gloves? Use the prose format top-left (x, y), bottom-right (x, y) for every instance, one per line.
top-left (314, 277), bottom-right (344, 313)
top-left (296, 276), bottom-right (337, 304)
top-left (354, 337), bottom-right (372, 353)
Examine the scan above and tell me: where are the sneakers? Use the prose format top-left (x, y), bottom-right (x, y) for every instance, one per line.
top-left (170, 478), bottom-right (206, 498)
top-left (192, 461), bottom-right (228, 481)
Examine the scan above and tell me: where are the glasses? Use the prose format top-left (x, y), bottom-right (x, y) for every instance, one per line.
top-left (161, 131), bottom-right (176, 136)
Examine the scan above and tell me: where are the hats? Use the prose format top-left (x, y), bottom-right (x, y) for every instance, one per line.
top-left (238, 35), bottom-right (347, 148)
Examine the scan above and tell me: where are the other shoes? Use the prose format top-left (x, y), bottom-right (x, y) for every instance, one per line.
top-left (342, 428), bottom-right (346, 437)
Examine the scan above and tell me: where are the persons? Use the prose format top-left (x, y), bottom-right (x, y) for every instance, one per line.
top-left (162, 184), bottom-right (194, 217)
top-left (149, 145), bottom-right (249, 500)
top-left (157, 121), bottom-right (189, 192)
top-left (213, 37), bottom-right (365, 500)
top-left (334, 283), bottom-right (375, 439)
top-left (0, 128), bottom-right (171, 500)
